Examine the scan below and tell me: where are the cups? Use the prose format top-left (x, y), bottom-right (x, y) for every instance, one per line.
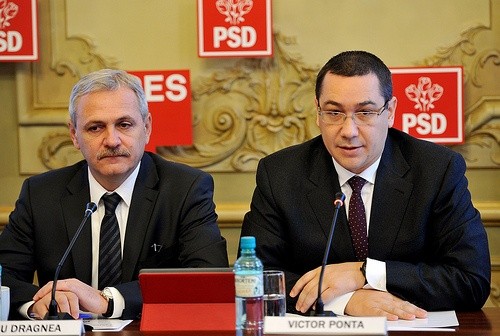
top-left (262, 270), bottom-right (286, 316)
top-left (0, 286), bottom-right (10, 321)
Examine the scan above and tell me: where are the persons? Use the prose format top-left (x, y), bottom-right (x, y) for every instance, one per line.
top-left (237, 51), bottom-right (491, 320)
top-left (0, 69), bottom-right (229, 321)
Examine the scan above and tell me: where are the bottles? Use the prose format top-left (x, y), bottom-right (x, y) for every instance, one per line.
top-left (235, 236), bottom-right (264, 330)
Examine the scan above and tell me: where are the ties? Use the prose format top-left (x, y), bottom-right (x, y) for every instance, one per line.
top-left (346, 176), bottom-right (370, 262)
top-left (96, 193), bottom-right (125, 289)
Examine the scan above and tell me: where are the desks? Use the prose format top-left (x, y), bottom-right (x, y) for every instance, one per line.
top-left (7, 307), bottom-right (500, 336)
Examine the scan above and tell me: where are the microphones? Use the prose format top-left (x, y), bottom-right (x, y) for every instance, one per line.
top-left (44, 202), bottom-right (97, 320)
top-left (309, 191), bottom-right (346, 316)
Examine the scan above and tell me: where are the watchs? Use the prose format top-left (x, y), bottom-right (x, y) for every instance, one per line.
top-left (100, 288), bottom-right (114, 317)
top-left (360, 261), bottom-right (368, 284)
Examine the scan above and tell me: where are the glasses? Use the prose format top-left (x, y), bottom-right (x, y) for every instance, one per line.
top-left (316, 99), bottom-right (389, 124)
